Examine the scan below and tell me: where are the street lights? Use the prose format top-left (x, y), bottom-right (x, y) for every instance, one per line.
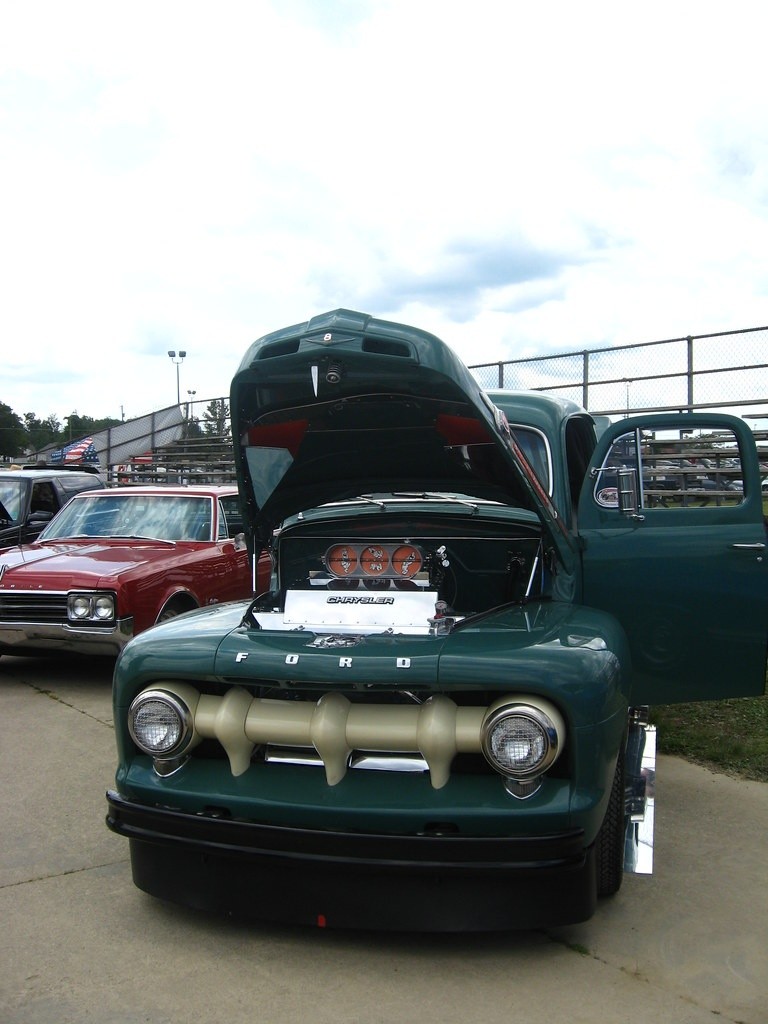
top-left (187, 390), bottom-right (196, 422)
top-left (168, 350), bottom-right (186, 404)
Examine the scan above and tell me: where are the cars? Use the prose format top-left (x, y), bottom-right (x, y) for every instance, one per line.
top-left (0, 465), bottom-right (109, 587)
top-left (0, 484), bottom-right (282, 679)
top-left (632, 453), bottom-right (768, 502)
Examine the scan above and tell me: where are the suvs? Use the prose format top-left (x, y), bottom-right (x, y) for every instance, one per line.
top-left (106, 307), bottom-right (768, 927)
